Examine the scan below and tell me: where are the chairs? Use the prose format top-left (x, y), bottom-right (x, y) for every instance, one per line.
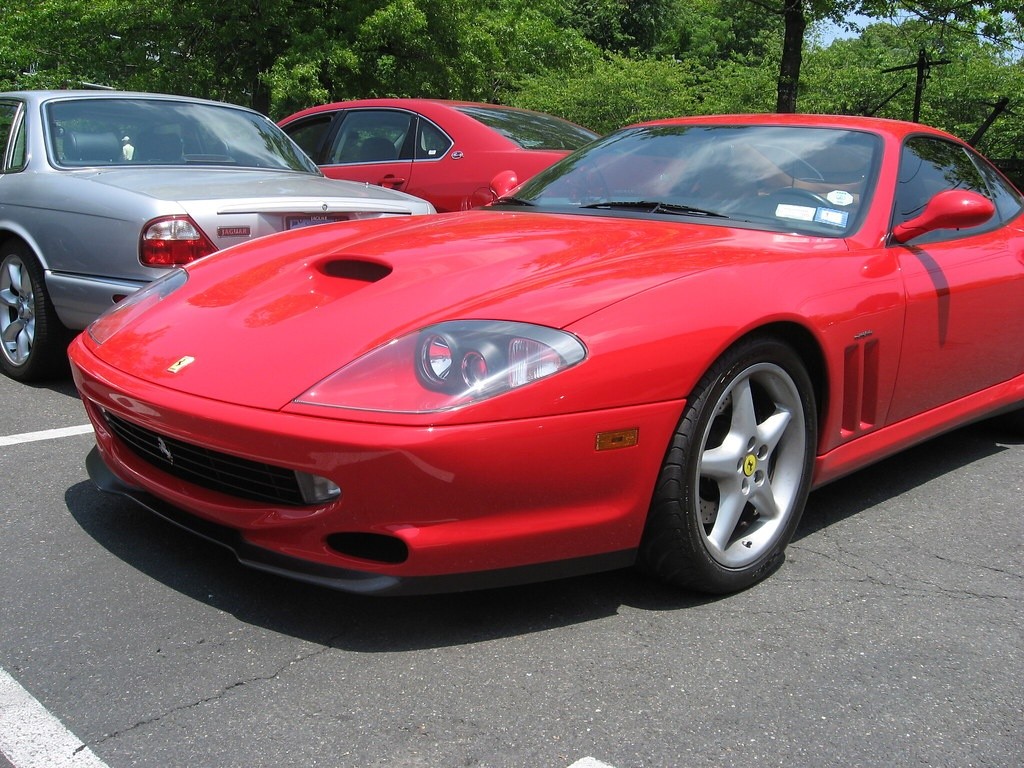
top-left (134, 132), bottom-right (182, 160)
top-left (357, 136), bottom-right (397, 161)
top-left (64, 126), bottom-right (121, 160)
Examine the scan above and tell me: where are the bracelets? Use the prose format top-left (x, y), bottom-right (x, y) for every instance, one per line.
top-left (791, 175), bottom-right (795, 187)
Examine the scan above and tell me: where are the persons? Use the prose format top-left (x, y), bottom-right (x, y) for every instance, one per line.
top-left (733, 130), bottom-right (873, 192)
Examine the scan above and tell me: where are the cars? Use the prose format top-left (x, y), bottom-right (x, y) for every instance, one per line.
top-left (0, 87), bottom-right (436, 383)
top-left (271, 97), bottom-right (605, 215)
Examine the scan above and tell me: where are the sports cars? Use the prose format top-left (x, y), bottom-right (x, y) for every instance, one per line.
top-left (66, 113), bottom-right (1024, 600)
